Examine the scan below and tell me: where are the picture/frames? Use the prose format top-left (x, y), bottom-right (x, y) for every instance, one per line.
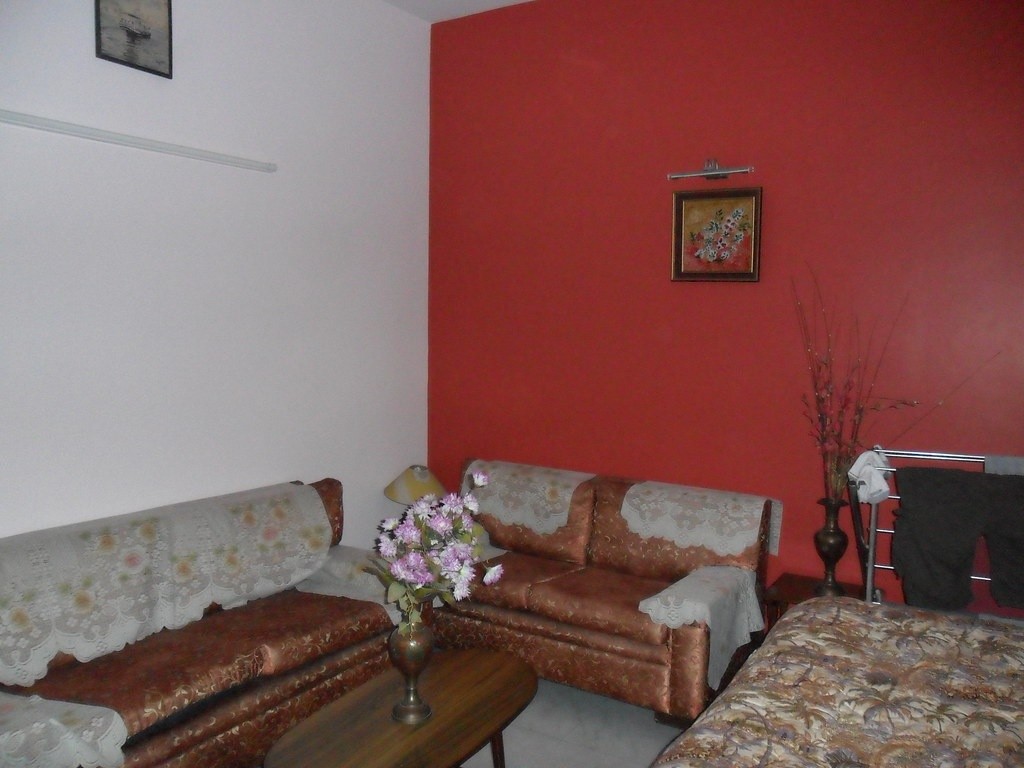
top-left (670, 186), bottom-right (761, 283)
top-left (94, 0), bottom-right (173, 79)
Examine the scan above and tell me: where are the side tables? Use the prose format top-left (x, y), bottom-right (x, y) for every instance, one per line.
top-left (759, 573), bottom-right (885, 639)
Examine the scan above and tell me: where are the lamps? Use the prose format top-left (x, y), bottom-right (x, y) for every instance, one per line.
top-left (383, 465), bottom-right (449, 507)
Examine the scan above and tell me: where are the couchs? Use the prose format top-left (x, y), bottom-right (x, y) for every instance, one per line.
top-left (427, 458), bottom-right (783, 730)
top-left (0, 478), bottom-right (429, 768)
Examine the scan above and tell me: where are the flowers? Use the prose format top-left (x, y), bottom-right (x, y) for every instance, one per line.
top-left (361, 471), bottom-right (506, 643)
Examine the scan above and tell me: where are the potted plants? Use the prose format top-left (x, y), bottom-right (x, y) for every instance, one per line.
top-left (788, 260), bottom-right (926, 598)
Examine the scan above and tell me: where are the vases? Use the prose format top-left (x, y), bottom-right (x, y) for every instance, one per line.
top-left (387, 607), bottom-right (432, 723)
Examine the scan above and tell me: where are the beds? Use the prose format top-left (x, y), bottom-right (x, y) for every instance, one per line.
top-left (634, 597), bottom-right (1024, 768)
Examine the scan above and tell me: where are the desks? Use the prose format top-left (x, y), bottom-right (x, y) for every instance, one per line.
top-left (261, 648), bottom-right (538, 768)
